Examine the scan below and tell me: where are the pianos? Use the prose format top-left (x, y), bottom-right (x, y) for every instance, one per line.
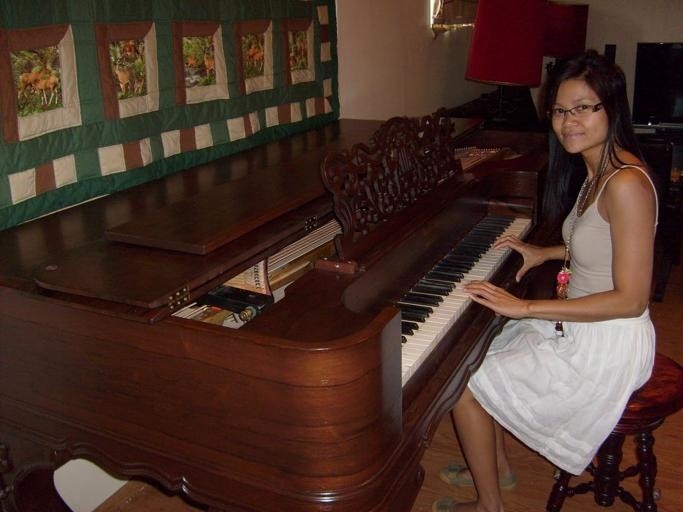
top-left (1, 107), bottom-right (549, 510)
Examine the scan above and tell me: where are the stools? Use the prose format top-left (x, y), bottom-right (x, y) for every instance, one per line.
top-left (545, 352), bottom-right (682, 510)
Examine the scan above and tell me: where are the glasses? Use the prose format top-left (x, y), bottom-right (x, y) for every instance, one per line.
top-left (543, 101), bottom-right (604, 123)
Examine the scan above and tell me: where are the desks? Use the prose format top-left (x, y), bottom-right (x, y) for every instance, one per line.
top-left (456, 128), bottom-right (552, 224)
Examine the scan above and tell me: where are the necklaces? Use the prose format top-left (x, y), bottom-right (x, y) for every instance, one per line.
top-left (552, 176), bottom-right (595, 336)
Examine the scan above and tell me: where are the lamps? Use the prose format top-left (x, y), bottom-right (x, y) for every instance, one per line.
top-left (464, 1), bottom-right (589, 132)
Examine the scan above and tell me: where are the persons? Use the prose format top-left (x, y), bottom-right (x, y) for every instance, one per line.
top-left (430, 47), bottom-right (659, 512)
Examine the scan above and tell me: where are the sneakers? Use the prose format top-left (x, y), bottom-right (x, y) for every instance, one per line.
top-left (431, 497), bottom-right (457, 512)
top-left (437, 460), bottom-right (519, 491)
